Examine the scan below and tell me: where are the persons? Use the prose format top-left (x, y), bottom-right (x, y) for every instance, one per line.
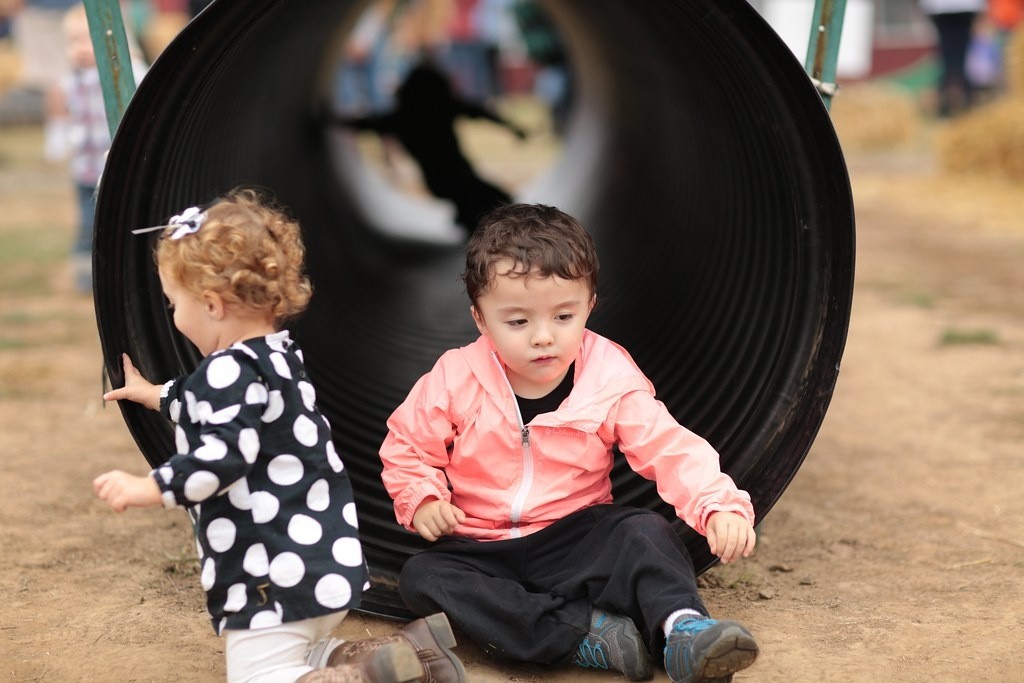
top-left (87, 189), bottom-right (470, 682)
top-left (378, 202), bottom-right (757, 683)
top-left (916, 0), bottom-right (1023, 120)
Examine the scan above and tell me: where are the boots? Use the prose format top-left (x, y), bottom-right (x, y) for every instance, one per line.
top-left (294, 641), bottom-right (426, 682)
top-left (325, 612), bottom-right (469, 683)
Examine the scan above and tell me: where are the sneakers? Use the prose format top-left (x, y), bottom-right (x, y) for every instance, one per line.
top-left (569, 606), bottom-right (653, 682)
top-left (665, 614), bottom-right (760, 683)
